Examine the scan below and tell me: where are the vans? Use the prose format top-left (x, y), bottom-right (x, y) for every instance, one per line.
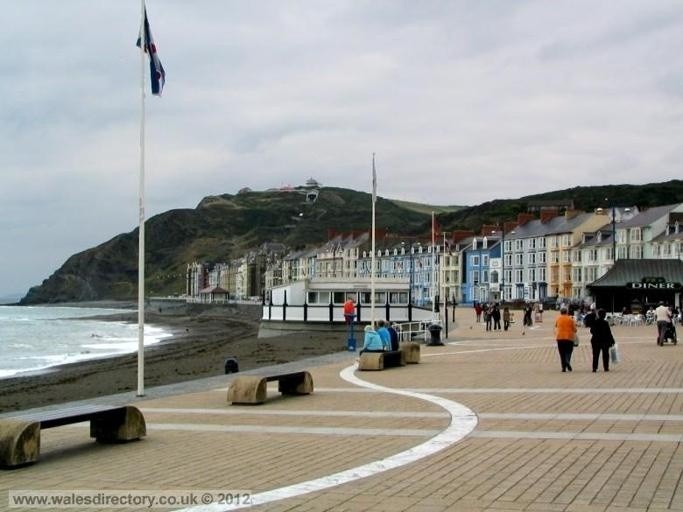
top-left (173, 292), bottom-right (178, 298)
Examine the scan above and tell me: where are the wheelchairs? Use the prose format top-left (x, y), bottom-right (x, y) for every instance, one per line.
top-left (656, 324), bottom-right (677, 346)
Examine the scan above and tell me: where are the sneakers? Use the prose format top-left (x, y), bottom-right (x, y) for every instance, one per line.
top-left (485, 326), bottom-right (509, 331)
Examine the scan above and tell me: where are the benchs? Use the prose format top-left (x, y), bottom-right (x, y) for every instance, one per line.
top-left (0, 403), bottom-right (146, 467)
top-left (358, 342), bottom-right (420, 370)
top-left (227, 369), bottom-right (314, 404)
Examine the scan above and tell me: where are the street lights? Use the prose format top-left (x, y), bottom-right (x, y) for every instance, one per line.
top-left (490, 228), bottom-right (515, 305)
top-left (401, 240), bottom-right (420, 307)
top-left (324, 246), bottom-right (343, 278)
top-left (272, 252), bottom-right (290, 285)
top-left (593, 205), bottom-right (632, 262)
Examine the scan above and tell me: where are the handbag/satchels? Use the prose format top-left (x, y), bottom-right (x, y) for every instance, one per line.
top-left (574, 335), bottom-right (578, 346)
top-left (610, 343), bottom-right (620, 364)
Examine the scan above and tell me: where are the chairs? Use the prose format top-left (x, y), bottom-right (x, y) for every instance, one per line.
top-left (573, 309), bottom-right (680, 328)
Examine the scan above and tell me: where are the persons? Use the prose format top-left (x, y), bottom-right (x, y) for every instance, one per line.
top-left (590, 309), bottom-right (615, 372)
top-left (359, 325), bottom-right (384, 356)
top-left (344, 297), bottom-right (356, 326)
top-left (474, 301), bottom-right (510, 331)
top-left (384, 320), bottom-right (399, 351)
top-left (224, 355), bottom-right (240, 375)
top-left (554, 308), bottom-right (577, 372)
top-left (645, 300), bottom-right (682, 347)
top-left (375, 320), bottom-right (392, 353)
top-left (524, 304), bottom-right (532, 326)
top-left (560, 300), bottom-right (598, 328)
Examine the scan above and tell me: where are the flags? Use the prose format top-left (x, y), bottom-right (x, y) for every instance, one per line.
top-left (433, 216), bottom-right (441, 243)
top-left (136, 5), bottom-right (165, 98)
